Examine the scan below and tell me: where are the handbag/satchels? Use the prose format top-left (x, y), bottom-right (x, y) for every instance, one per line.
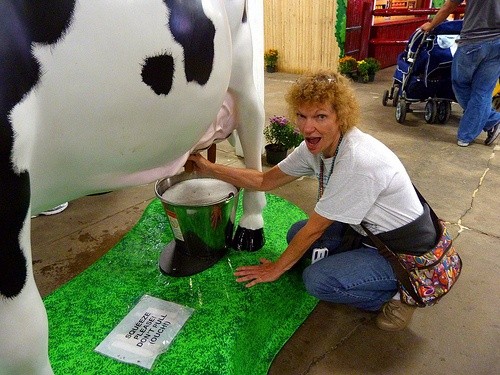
top-left (395, 219), bottom-right (462, 308)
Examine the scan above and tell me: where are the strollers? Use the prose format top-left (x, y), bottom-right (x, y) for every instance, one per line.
top-left (382, 18), bottom-right (458, 124)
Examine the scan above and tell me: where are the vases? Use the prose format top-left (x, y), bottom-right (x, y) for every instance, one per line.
top-left (264, 143), bottom-right (288, 165)
top-left (366, 71), bottom-right (376, 83)
top-left (352, 73), bottom-right (364, 83)
top-left (266, 64), bottom-right (276, 72)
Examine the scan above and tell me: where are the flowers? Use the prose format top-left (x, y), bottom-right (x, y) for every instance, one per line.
top-left (337, 55), bottom-right (381, 83)
top-left (263, 115), bottom-right (304, 150)
top-left (264, 49), bottom-right (280, 67)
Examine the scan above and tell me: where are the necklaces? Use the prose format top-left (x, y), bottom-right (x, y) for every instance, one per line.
top-left (318, 132), bottom-right (345, 203)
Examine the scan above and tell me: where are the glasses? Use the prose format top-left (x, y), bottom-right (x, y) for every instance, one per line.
top-left (296, 76), bottom-right (336, 96)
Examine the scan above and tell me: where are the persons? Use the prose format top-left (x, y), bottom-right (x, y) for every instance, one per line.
top-left (188, 69), bottom-right (437, 332)
top-left (421, 0), bottom-right (500, 147)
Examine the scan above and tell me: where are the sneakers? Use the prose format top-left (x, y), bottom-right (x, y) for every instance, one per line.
top-left (457, 139), bottom-right (473, 146)
top-left (375, 298), bottom-right (417, 331)
top-left (485, 122), bottom-right (500, 145)
